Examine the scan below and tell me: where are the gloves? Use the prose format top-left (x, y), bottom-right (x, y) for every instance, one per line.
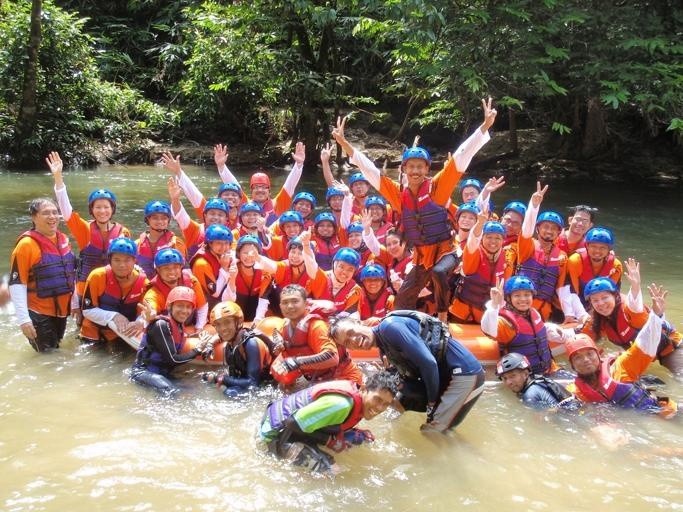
top-left (270, 356), bottom-right (303, 386)
top-left (323, 429), bottom-right (374, 453)
top-left (193, 330), bottom-right (215, 364)
top-left (197, 371), bottom-right (225, 390)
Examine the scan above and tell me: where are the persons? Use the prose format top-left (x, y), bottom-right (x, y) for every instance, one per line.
top-left (41, 140), bottom-right (683, 421)
top-left (0, 272), bottom-right (11, 310)
top-left (7, 197), bottom-right (82, 354)
top-left (253, 370), bottom-right (403, 482)
top-left (327, 308), bottom-right (490, 457)
top-left (329, 95), bottom-right (498, 326)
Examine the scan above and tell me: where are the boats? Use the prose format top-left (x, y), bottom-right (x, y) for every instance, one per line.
top-left (173, 310), bottom-right (576, 369)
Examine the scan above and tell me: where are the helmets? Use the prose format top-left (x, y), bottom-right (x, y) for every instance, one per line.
top-left (151, 248), bottom-right (182, 268)
top-left (143, 200), bottom-right (171, 221)
top-left (279, 173), bottom-right (387, 280)
top-left (583, 277), bottom-right (618, 301)
top-left (87, 189), bottom-right (116, 210)
top-left (165, 286), bottom-right (198, 311)
top-left (399, 148), bottom-right (431, 165)
top-left (585, 227), bottom-right (614, 245)
top-left (106, 237), bottom-right (137, 258)
top-left (202, 172), bottom-right (270, 253)
top-left (564, 333), bottom-right (599, 361)
top-left (209, 301), bottom-right (245, 326)
top-left (503, 274), bottom-right (537, 297)
top-left (454, 178), bottom-right (563, 238)
